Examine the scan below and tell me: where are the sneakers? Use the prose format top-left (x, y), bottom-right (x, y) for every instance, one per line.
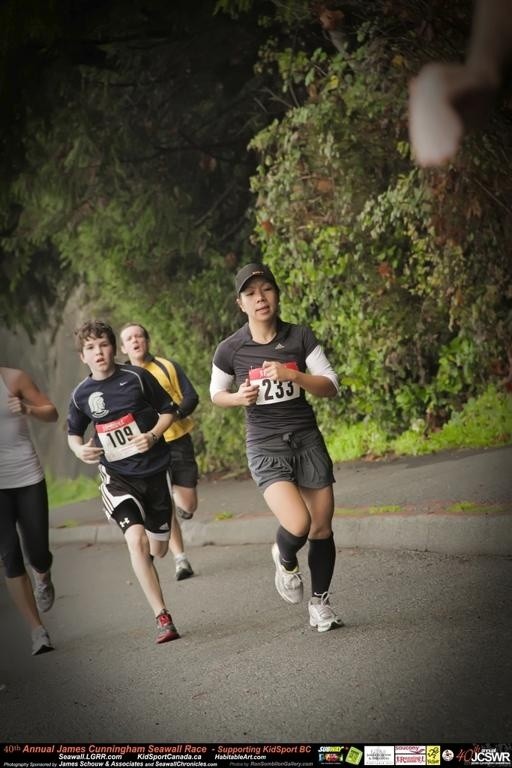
top-left (271, 543), bottom-right (303, 604)
top-left (31, 627), bottom-right (54, 655)
top-left (155, 614), bottom-right (178, 644)
top-left (308, 592), bottom-right (344, 632)
top-left (176, 507), bottom-right (193, 520)
top-left (32, 571), bottom-right (55, 613)
top-left (175, 559), bottom-right (194, 581)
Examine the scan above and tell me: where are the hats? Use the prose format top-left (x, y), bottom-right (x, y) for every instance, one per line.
top-left (235, 263), bottom-right (278, 298)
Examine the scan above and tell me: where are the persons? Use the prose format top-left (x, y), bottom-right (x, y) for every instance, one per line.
top-left (117, 321), bottom-right (200, 579)
top-left (67, 320), bottom-right (179, 644)
top-left (209, 264), bottom-right (343, 633)
top-left (0, 366), bottom-right (59, 655)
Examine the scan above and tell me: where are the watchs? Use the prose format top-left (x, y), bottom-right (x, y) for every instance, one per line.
top-left (148, 431), bottom-right (159, 444)
